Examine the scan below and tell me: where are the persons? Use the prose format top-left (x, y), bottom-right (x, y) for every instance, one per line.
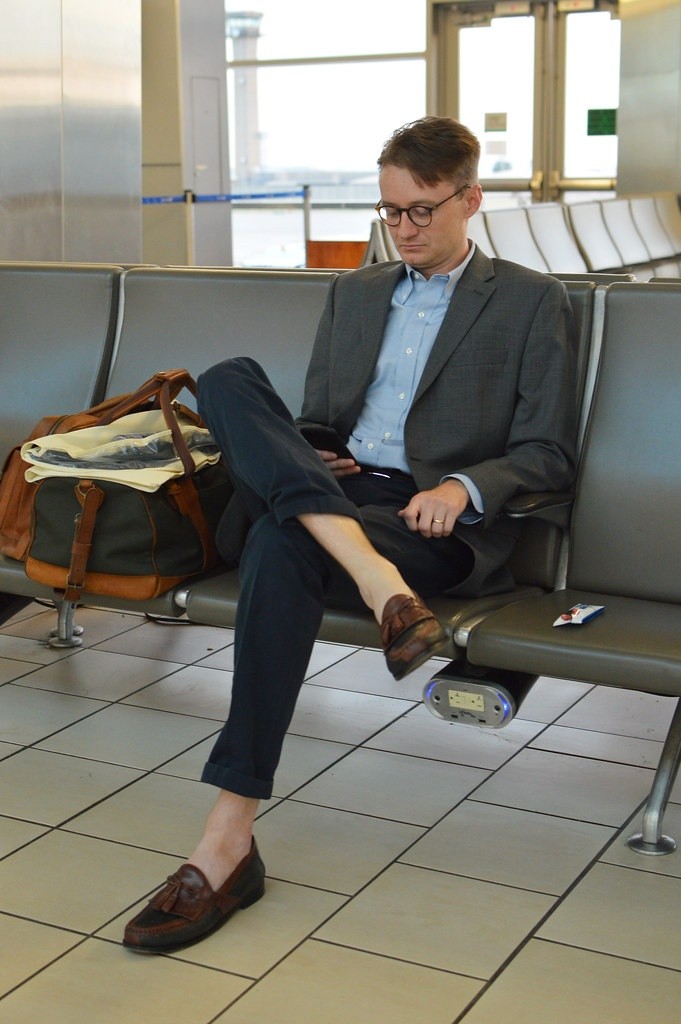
top-left (123, 114), bottom-right (580, 957)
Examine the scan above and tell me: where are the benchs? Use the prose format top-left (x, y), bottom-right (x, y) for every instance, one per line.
top-left (362, 189), bottom-right (681, 284)
top-left (1, 258), bottom-right (681, 854)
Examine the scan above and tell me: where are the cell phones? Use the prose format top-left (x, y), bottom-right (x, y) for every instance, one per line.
top-left (300, 427), bottom-right (359, 466)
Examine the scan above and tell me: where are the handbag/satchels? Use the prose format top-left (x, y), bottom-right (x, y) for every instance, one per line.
top-left (0, 370), bottom-right (245, 607)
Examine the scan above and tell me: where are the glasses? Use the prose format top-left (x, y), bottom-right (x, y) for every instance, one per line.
top-left (374, 184), bottom-right (472, 228)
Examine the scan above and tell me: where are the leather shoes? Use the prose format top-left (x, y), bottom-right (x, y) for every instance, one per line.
top-left (379, 585), bottom-right (448, 681)
top-left (121, 832), bottom-right (265, 955)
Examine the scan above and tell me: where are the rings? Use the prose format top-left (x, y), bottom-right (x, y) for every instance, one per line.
top-left (433, 519), bottom-right (443, 524)
